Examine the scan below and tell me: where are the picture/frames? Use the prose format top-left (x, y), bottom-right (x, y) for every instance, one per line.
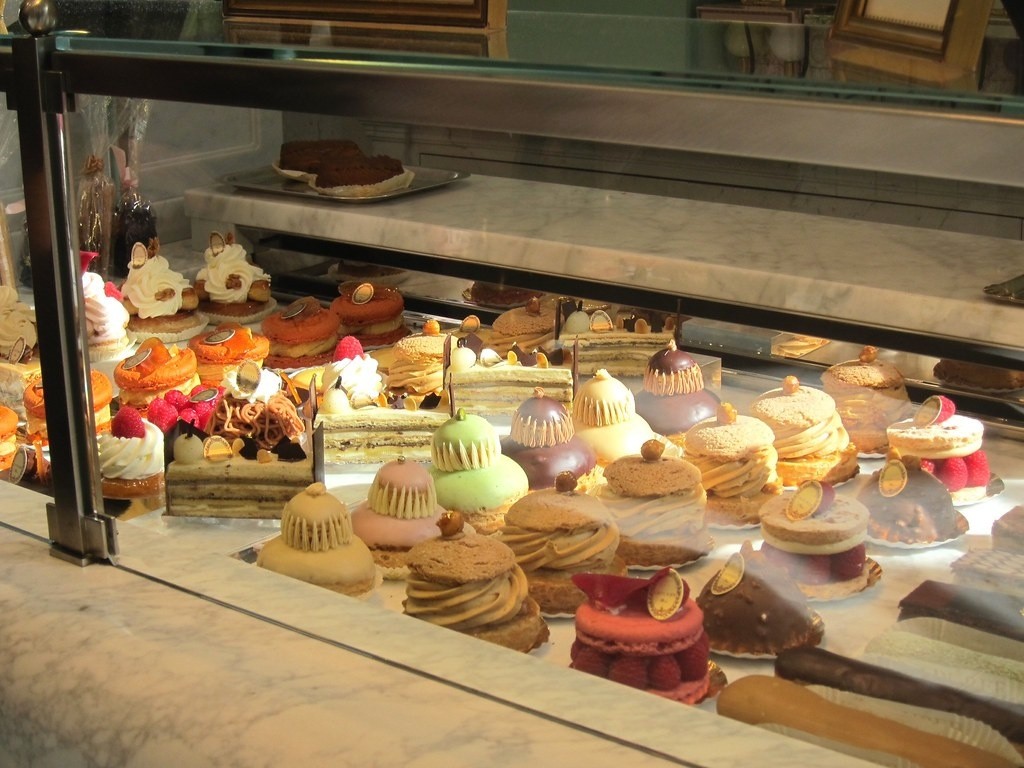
top-left (220, 0), bottom-right (507, 33)
top-left (832, 0), bottom-right (994, 72)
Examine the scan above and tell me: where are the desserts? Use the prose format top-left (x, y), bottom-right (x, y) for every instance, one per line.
top-left (0, 235), bottom-right (991, 706)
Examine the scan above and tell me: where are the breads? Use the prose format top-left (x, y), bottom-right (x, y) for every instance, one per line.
top-left (714, 504), bottom-right (1024, 768)
top-left (271, 139), bottom-right (414, 198)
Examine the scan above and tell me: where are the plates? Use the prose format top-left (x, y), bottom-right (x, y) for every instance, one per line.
top-left (982, 273), bottom-right (1024, 305)
top-left (0, 296), bottom-right (1005, 709)
